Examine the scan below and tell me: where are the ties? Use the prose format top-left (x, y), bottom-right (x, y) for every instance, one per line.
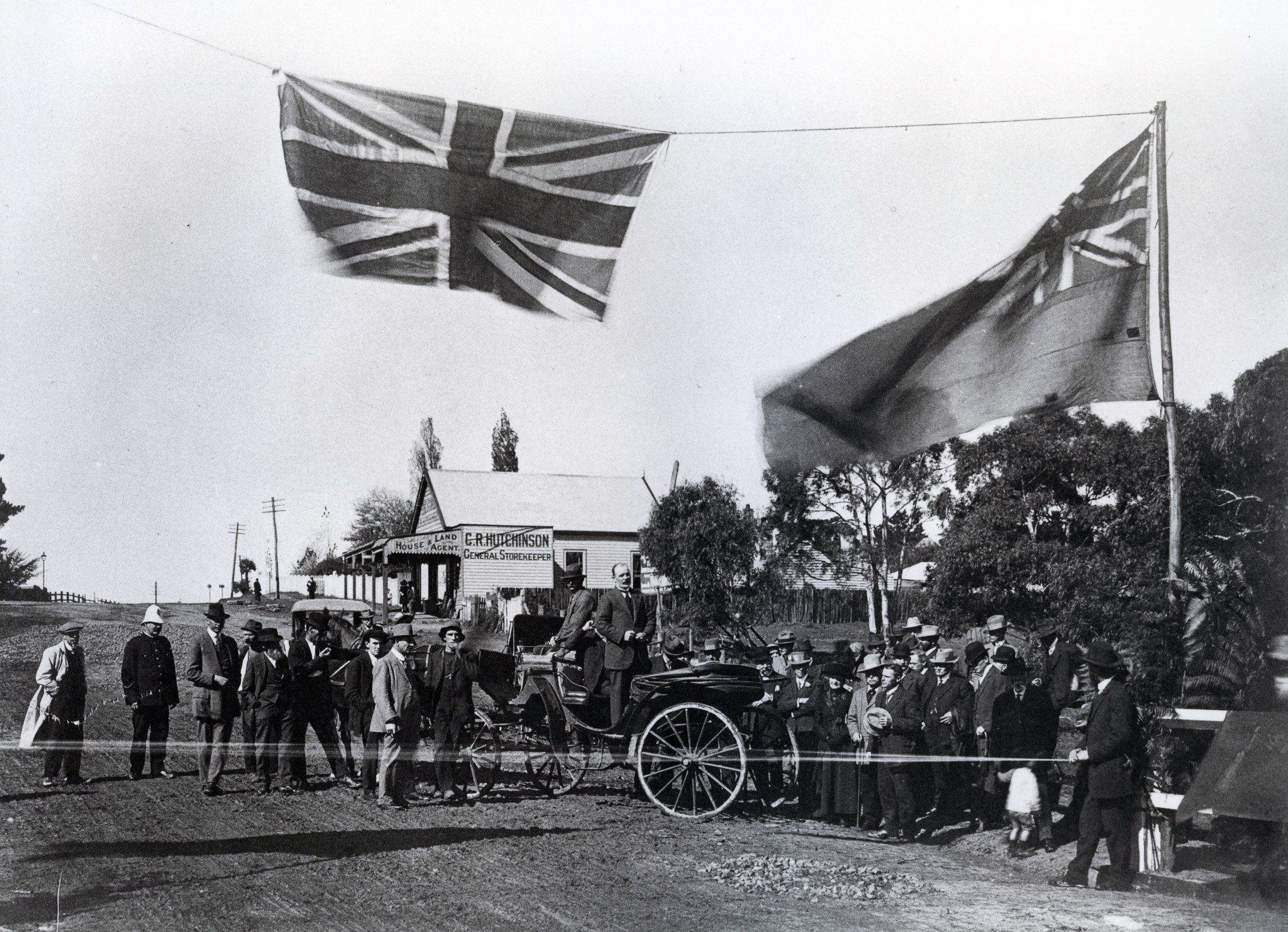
top-left (939, 679), bottom-right (943, 686)
top-left (885, 694), bottom-right (891, 704)
top-left (619, 589), bottom-right (631, 606)
top-left (1017, 694), bottom-right (1021, 702)
top-left (978, 681), bottom-right (980, 688)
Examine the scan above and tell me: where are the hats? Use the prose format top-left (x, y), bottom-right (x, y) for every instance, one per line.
top-left (863, 707), bottom-right (893, 736)
top-left (889, 624), bottom-right (909, 636)
top-left (1000, 657), bottom-right (1032, 677)
top-left (867, 630), bottom-right (886, 651)
top-left (901, 634), bottom-right (920, 649)
top-left (902, 617), bottom-right (924, 628)
top-left (1032, 620), bottom-right (1060, 639)
top-left (964, 641), bottom-right (990, 664)
top-left (1080, 641), bottom-right (1124, 666)
top-left (990, 645), bottom-right (1016, 662)
top-left (663, 629), bottom-right (864, 677)
top-left (560, 564), bottom-right (587, 580)
top-left (984, 614), bottom-right (1010, 631)
top-left (929, 648), bottom-right (959, 663)
top-left (889, 643), bottom-right (914, 656)
top-left (56, 602), bottom-right (466, 652)
top-left (857, 654), bottom-right (886, 673)
top-left (917, 625), bottom-right (944, 637)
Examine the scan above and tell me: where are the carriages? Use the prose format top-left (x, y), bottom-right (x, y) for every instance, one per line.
top-left (289, 599), bottom-right (805, 821)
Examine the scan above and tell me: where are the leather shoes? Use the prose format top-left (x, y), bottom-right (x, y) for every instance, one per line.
top-left (41, 762), bottom-right (465, 808)
top-left (754, 791), bottom-right (1127, 891)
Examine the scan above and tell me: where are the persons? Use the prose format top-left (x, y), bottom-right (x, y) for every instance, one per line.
top-left (254, 578), bottom-right (261, 601)
top-left (647, 614), bottom-right (1097, 859)
top-left (550, 564), bottom-right (597, 647)
top-left (281, 637), bottom-right (384, 784)
top-left (422, 622), bottom-right (482, 800)
top-left (1048, 641), bottom-right (1137, 887)
top-left (565, 562), bottom-right (656, 725)
top-left (287, 614), bottom-right (363, 794)
top-left (398, 580), bottom-right (409, 613)
top-left (406, 581), bottom-right (417, 616)
top-left (238, 619), bottom-right (265, 775)
top-left (307, 577), bottom-right (316, 599)
top-left (371, 623), bottom-right (430, 809)
top-left (243, 627), bottom-right (290, 793)
top-left (344, 630), bottom-right (389, 799)
top-left (19, 622), bottom-right (88, 786)
top-left (185, 602), bottom-right (241, 793)
top-left (357, 610), bottom-right (375, 633)
top-left (121, 604), bottom-right (180, 780)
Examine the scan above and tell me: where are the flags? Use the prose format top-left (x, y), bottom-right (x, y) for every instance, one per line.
top-left (272, 66), bottom-right (671, 322)
top-left (760, 114), bottom-right (1160, 472)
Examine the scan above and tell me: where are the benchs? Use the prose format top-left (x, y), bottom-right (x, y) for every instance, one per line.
top-left (512, 613), bottom-right (566, 668)
top-left (551, 652), bottom-right (611, 709)
